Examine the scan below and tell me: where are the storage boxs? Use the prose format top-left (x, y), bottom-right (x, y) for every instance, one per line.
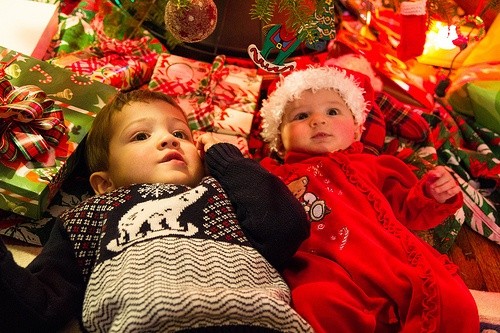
top-left (44, 36), bottom-right (262, 158)
top-left (0, 46), bottom-right (121, 220)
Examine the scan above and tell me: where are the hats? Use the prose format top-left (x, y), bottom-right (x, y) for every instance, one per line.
top-left (259, 63), bottom-right (375, 154)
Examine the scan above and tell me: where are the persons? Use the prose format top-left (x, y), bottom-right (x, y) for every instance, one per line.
top-left (256, 65), bottom-right (482, 332)
top-left (0, 89), bottom-right (313, 333)
